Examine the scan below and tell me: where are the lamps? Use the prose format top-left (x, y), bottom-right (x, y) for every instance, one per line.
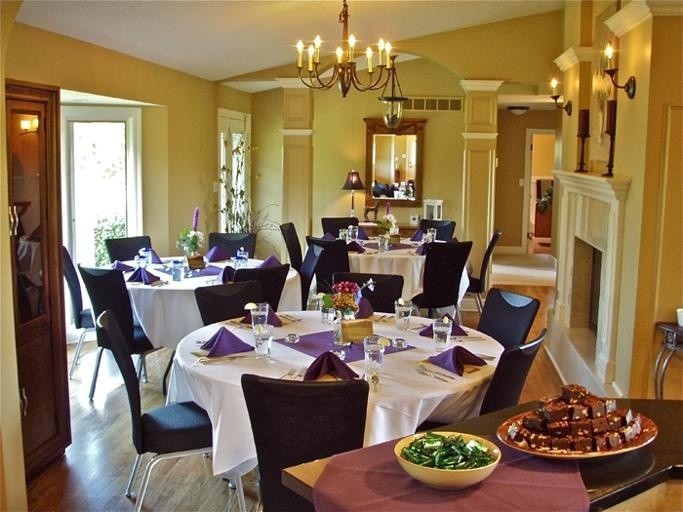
top-left (600, 43), bottom-right (636, 103)
top-left (17, 118), bottom-right (38, 135)
top-left (377, 55), bottom-right (407, 130)
top-left (292, 2), bottom-right (394, 95)
top-left (341, 169), bottom-right (365, 216)
top-left (545, 76), bottom-right (572, 118)
top-left (507, 105), bottom-right (531, 118)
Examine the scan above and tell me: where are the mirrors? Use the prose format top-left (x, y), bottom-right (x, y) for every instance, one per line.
top-left (363, 116), bottom-right (427, 207)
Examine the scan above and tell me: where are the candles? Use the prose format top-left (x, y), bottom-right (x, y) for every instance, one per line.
top-left (307, 43), bottom-right (314, 70)
top-left (605, 44), bottom-right (614, 69)
top-left (549, 78), bottom-right (558, 96)
top-left (335, 46), bottom-right (343, 62)
top-left (366, 46), bottom-right (374, 74)
top-left (385, 41), bottom-right (391, 66)
top-left (348, 31), bottom-right (354, 61)
top-left (296, 40), bottom-right (303, 68)
top-left (312, 34), bottom-right (322, 64)
top-left (377, 38), bottom-right (383, 64)
top-left (579, 108), bottom-right (589, 131)
top-left (607, 98), bottom-right (618, 134)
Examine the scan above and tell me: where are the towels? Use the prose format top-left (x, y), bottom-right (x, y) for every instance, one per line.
top-left (356, 299), bottom-right (373, 318)
top-left (410, 229), bottom-right (423, 241)
top-left (239, 305), bottom-right (281, 329)
top-left (112, 260), bottom-right (134, 271)
top-left (417, 243), bottom-right (427, 255)
top-left (128, 266), bottom-right (160, 284)
top-left (419, 313), bottom-right (467, 338)
top-left (199, 327), bottom-right (254, 357)
top-left (430, 345), bottom-right (486, 376)
top-left (260, 255), bottom-right (281, 267)
top-left (205, 246), bottom-right (230, 262)
top-left (359, 229), bottom-right (369, 240)
top-left (151, 250), bottom-right (160, 263)
top-left (302, 350), bottom-right (358, 382)
top-left (347, 241), bottom-right (364, 253)
top-left (321, 232), bottom-right (336, 240)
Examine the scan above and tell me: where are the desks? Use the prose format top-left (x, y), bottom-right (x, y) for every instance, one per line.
top-left (17, 234), bottom-right (44, 287)
top-left (654, 322), bottom-right (683, 398)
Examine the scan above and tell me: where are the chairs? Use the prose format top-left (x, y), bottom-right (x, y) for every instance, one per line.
top-left (95, 309), bottom-right (244, 511)
top-left (476, 288), bottom-right (540, 350)
top-left (478, 330), bottom-right (548, 414)
top-left (467, 231), bottom-right (502, 316)
top-left (234, 264), bottom-right (290, 312)
top-left (321, 218), bottom-right (358, 238)
top-left (61, 246), bottom-right (94, 380)
top-left (279, 221), bottom-right (303, 271)
top-left (239, 374), bottom-right (368, 511)
top-left (209, 231), bottom-right (256, 261)
top-left (77, 264), bottom-right (152, 401)
top-left (195, 281), bottom-right (263, 325)
top-left (105, 237), bottom-right (152, 264)
top-left (299, 244), bottom-right (327, 312)
top-left (306, 236), bottom-right (350, 293)
top-left (332, 271), bottom-right (404, 312)
top-left (412, 241), bottom-right (474, 325)
top-left (420, 218), bottom-right (456, 241)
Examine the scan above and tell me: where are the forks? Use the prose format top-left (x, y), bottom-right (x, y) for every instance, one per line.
top-left (278, 368), bottom-right (294, 380)
top-left (412, 366), bottom-right (449, 384)
top-left (417, 362), bottom-right (456, 381)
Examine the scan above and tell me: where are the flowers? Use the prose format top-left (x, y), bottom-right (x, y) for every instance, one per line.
top-left (328, 281), bottom-right (358, 339)
top-left (176, 206), bottom-right (205, 254)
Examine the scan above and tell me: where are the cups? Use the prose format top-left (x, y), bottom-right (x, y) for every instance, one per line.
top-left (171, 259), bottom-right (184, 282)
top-left (249, 302), bottom-right (270, 329)
top-left (426, 226), bottom-right (438, 243)
top-left (378, 234), bottom-right (387, 255)
top-left (134, 251), bottom-right (153, 271)
top-left (338, 226), bottom-right (359, 244)
top-left (394, 299), bottom-right (412, 329)
top-left (252, 323), bottom-right (274, 357)
top-left (318, 297), bottom-right (335, 323)
top-left (432, 317), bottom-right (453, 351)
top-left (236, 250), bottom-right (248, 269)
top-left (363, 334), bottom-right (385, 385)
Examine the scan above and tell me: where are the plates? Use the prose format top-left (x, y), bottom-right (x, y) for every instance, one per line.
top-left (394, 429), bottom-right (502, 491)
top-left (496, 407), bottom-right (658, 460)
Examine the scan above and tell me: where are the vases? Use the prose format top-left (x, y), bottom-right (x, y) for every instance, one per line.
top-left (332, 311), bottom-right (354, 345)
top-left (183, 249), bottom-right (197, 262)
